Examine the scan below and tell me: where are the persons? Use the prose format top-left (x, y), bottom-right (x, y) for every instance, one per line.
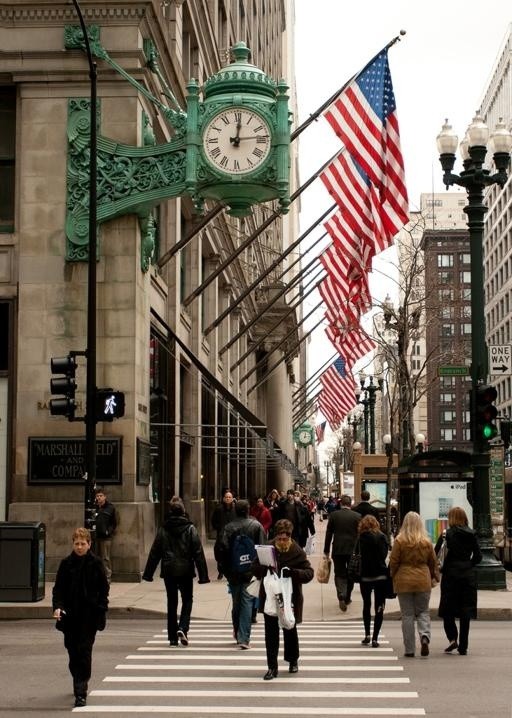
top-left (142, 496), bottom-right (210, 647)
top-left (52, 527), bottom-right (110, 706)
top-left (212, 487), bottom-right (391, 680)
top-left (435, 507), bottom-right (483, 655)
top-left (390, 511), bottom-right (439, 657)
top-left (95, 488), bottom-right (116, 583)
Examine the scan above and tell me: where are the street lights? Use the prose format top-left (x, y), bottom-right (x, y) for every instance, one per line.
top-left (434, 107), bottom-right (512, 592)
top-left (340, 293), bottom-right (426, 482)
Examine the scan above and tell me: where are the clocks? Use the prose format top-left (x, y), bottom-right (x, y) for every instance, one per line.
top-left (300, 430), bottom-right (311, 444)
top-left (202, 107), bottom-right (272, 175)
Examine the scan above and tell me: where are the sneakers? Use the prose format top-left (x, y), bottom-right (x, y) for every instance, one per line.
top-left (170, 640), bottom-right (178, 647)
top-left (339, 599), bottom-right (352, 611)
top-left (445, 640), bottom-right (466, 655)
top-left (421, 637), bottom-right (429, 656)
top-left (404, 654), bottom-right (414, 657)
top-left (362, 637), bottom-right (370, 643)
top-left (233, 631), bottom-right (251, 649)
top-left (177, 629), bottom-right (188, 646)
top-left (372, 642), bottom-right (379, 647)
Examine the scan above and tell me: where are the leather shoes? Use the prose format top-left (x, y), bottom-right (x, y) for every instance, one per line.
top-left (264, 668), bottom-right (277, 680)
top-left (289, 660), bottom-right (298, 672)
top-left (74, 691), bottom-right (86, 705)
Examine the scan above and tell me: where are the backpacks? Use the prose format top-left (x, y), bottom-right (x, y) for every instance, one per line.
top-left (224, 520), bottom-right (258, 574)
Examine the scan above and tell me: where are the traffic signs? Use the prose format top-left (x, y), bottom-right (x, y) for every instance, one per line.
top-left (488, 344), bottom-right (512, 377)
top-left (434, 364), bottom-right (471, 377)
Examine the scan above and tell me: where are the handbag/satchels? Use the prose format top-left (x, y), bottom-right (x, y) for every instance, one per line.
top-left (348, 552), bottom-right (361, 582)
top-left (436, 529), bottom-right (449, 570)
top-left (264, 567), bottom-right (296, 630)
top-left (316, 554), bottom-right (331, 583)
top-left (384, 561), bottom-right (397, 598)
top-left (246, 578), bottom-right (261, 598)
top-left (305, 535), bottom-right (315, 555)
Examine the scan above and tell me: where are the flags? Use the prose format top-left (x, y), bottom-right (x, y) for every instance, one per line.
top-left (315, 148), bottom-right (395, 444)
top-left (319, 51), bottom-right (410, 241)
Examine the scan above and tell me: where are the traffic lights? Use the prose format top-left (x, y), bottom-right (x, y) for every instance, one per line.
top-left (49, 354), bottom-right (78, 419)
top-left (95, 390), bottom-right (126, 421)
top-left (473, 382), bottom-right (499, 444)
